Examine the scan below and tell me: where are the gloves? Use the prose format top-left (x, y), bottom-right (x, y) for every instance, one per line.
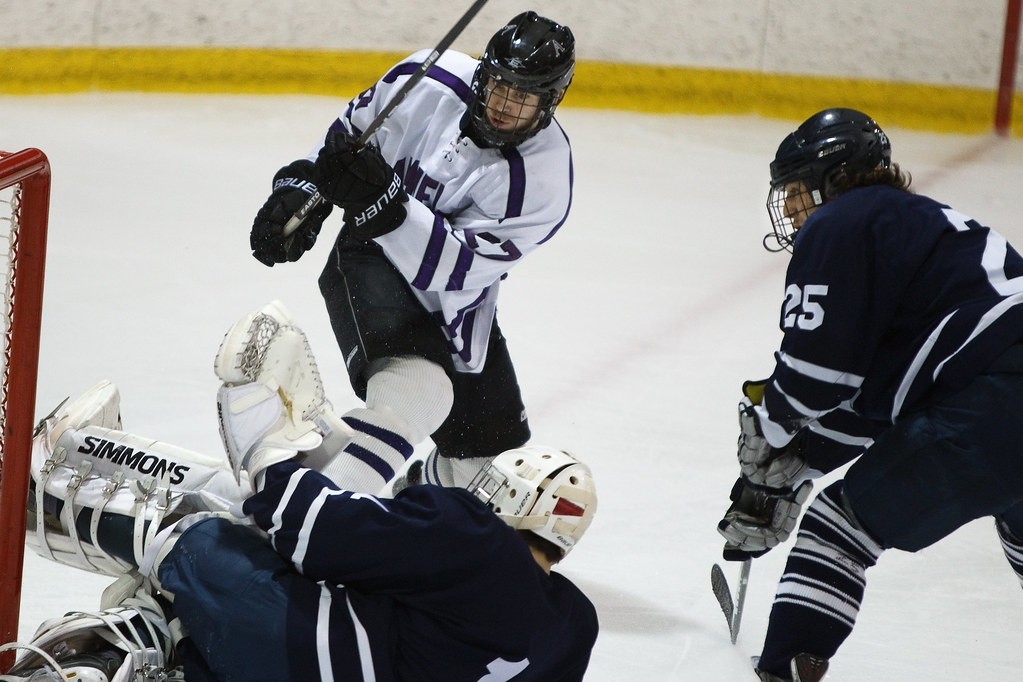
top-left (316, 129), bottom-right (409, 239)
top-left (713, 401), bottom-right (827, 564)
top-left (248, 158), bottom-right (333, 267)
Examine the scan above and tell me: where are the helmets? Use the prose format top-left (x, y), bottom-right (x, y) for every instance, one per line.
top-left (765, 106), bottom-right (892, 256)
top-left (467, 445), bottom-right (597, 556)
top-left (468, 10), bottom-right (576, 150)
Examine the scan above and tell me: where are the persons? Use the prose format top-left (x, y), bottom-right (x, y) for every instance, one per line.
top-left (251, 11), bottom-right (576, 497)
top-left (717, 108), bottom-right (1023, 682)
top-left (1, 306), bottom-right (599, 682)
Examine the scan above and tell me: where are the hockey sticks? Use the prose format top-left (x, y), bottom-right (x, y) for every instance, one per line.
top-left (283, 1), bottom-right (488, 237)
top-left (709, 556), bottom-right (753, 645)
